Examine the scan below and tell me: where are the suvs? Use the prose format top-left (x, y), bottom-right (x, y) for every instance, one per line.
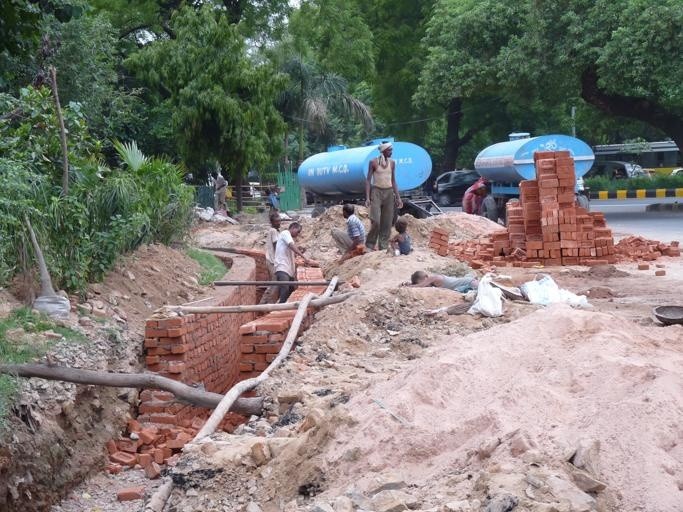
top-left (583, 161), bottom-right (646, 180)
top-left (230, 182), bottom-right (266, 199)
top-left (431, 168), bottom-right (484, 205)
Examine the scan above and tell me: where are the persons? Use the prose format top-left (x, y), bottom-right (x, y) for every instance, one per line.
top-left (331, 204), bottom-right (367, 263)
top-left (210, 171), bottom-right (230, 214)
top-left (402, 271), bottom-right (479, 291)
top-left (257, 214), bottom-right (281, 316)
top-left (388, 217), bottom-right (411, 256)
top-left (365, 141), bottom-right (403, 251)
top-left (265, 186), bottom-right (279, 210)
top-left (275, 223), bottom-right (317, 304)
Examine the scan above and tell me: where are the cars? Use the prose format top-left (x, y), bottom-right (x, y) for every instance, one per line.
top-left (667, 167), bottom-right (682, 179)
top-left (642, 167), bottom-right (655, 179)
